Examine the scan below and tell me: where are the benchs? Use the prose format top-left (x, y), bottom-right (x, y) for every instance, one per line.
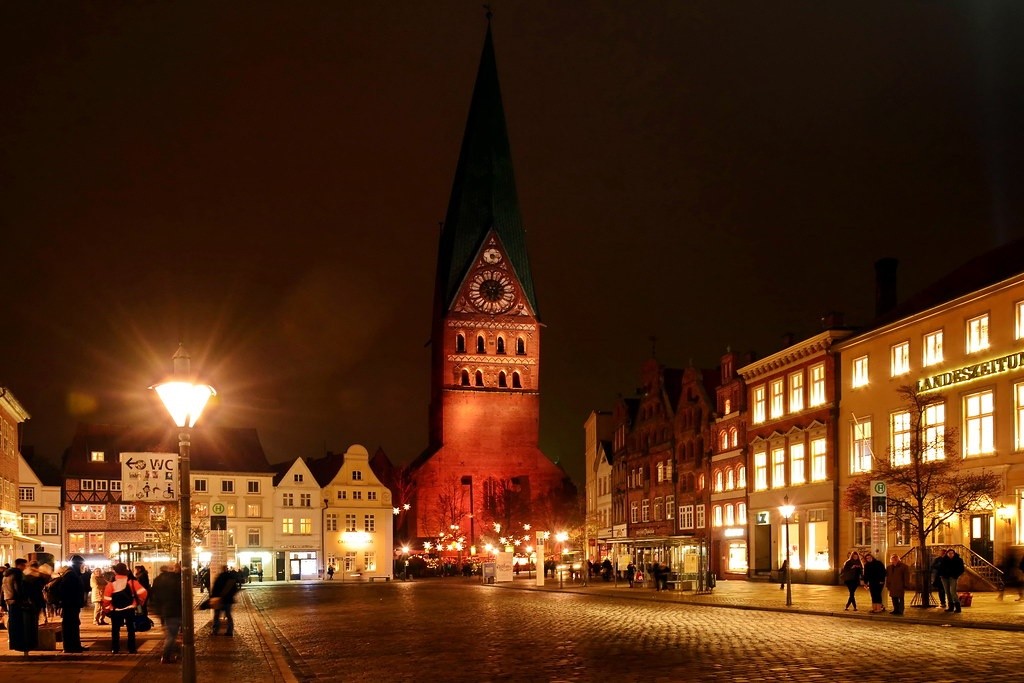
top-left (368, 574), bottom-right (391, 584)
top-left (667, 578), bottom-right (697, 591)
top-left (35, 621), bottom-right (65, 652)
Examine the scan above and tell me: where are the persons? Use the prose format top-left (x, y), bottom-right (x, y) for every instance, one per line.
top-left (863, 554), bottom-right (885, 612)
top-left (328, 565), bottom-right (334, 580)
top-left (842, 551), bottom-right (863, 611)
top-left (778, 560), bottom-right (787, 590)
top-left (992, 546), bottom-right (1024, 602)
top-left (627, 560), bottom-right (670, 592)
top-left (940, 549), bottom-right (965, 613)
top-left (515, 562), bottom-right (520, 574)
top-left (209, 565), bottom-right (236, 638)
top-left (543, 556), bottom-right (612, 581)
top-left (0, 553), bottom-right (182, 664)
top-left (440, 559), bottom-right (477, 576)
top-left (197, 565), bottom-right (264, 612)
top-left (885, 553), bottom-right (907, 616)
top-left (931, 549), bottom-right (948, 608)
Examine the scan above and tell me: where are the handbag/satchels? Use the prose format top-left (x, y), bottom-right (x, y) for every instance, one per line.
top-left (840, 568), bottom-right (852, 580)
top-left (959, 592), bottom-right (972, 606)
top-left (210, 597), bottom-right (221, 610)
top-left (134, 614), bottom-right (151, 632)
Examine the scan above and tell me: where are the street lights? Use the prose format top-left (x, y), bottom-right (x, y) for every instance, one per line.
top-left (485, 543), bottom-right (493, 562)
top-left (526, 545), bottom-right (532, 579)
top-left (145, 341), bottom-right (218, 683)
top-left (193, 536), bottom-right (202, 586)
top-left (555, 532), bottom-right (564, 590)
top-left (776, 493), bottom-right (796, 607)
top-left (459, 474), bottom-right (474, 546)
top-left (402, 545), bottom-right (409, 581)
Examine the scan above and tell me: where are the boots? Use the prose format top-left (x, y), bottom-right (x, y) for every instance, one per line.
top-left (872, 603), bottom-right (882, 613)
top-left (945, 602), bottom-right (953, 612)
top-left (954, 602), bottom-right (961, 613)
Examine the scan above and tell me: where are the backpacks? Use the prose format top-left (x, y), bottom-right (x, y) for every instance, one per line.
top-left (42, 569), bottom-right (78, 603)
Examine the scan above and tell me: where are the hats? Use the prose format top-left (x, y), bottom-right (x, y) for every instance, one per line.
top-left (113, 563), bottom-right (128, 575)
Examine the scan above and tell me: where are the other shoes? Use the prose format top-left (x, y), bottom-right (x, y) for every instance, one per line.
top-left (128, 648), bottom-right (137, 653)
top-left (891, 610), bottom-right (899, 614)
top-left (853, 605), bottom-right (856, 611)
top-left (209, 628), bottom-right (219, 635)
top-left (99, 621), bottom-right (108, 624)
top-left (845, 605), bottom-right (850, 611)
top-left (112, 649), bottom-right (118, 653)
top-left (62, 644), bottom-right (90, 653)
top-left (224, 632), bottom-right (233, 636)
top-left (161, 656), bottom-right (175, 662)
top-left (940, 604), bottom-right (946, 607)
